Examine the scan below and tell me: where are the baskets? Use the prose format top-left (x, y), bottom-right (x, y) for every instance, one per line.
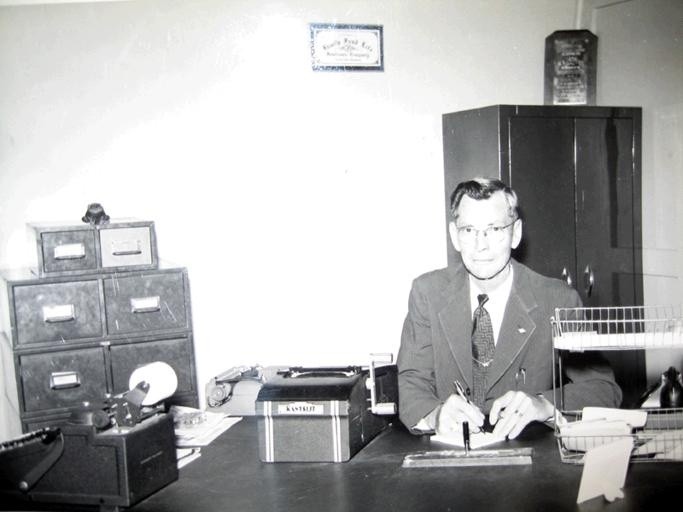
top-left (548, 302), bottom-right (683, 356)
top-left (548, 406), bottom-right (683, 464)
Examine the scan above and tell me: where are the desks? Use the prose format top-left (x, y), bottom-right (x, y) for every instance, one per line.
top-left (119, 405), bottom-right (681, 512)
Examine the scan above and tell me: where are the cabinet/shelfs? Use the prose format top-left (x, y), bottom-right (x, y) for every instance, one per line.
top-left (0, 215), bottom-right (202, 435)
top-left (546, 302), bottom-right (682, 468)
top-left (440, 100), bottom-right (647, 412)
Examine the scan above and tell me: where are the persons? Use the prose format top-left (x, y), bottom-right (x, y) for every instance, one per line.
top-left (396, 176), bottom-right (624, 442)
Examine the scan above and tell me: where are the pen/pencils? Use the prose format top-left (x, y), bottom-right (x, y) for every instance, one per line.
top-left (453, 380), bottom-right (489, 437)
top-left (520, 368), bottom-right (526, 384)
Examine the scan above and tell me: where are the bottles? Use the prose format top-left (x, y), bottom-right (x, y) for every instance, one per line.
top-left (660, 365), bottom-right (683, 413)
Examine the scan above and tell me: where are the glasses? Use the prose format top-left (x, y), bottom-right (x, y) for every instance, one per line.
top-left (453, 216), bottom-right (518, 244)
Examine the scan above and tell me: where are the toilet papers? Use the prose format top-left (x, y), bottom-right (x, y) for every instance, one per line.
top-left (128, 362), bottom-right (180, 407)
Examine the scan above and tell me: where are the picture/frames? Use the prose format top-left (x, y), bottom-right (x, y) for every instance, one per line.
top-left (308, 22), bottom-right (385, 75)
top-left (541, 27), bottom-right (599, 105)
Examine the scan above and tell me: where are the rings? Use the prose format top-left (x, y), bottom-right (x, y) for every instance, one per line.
top-left (515, 409), bottom-right (522, 417)
